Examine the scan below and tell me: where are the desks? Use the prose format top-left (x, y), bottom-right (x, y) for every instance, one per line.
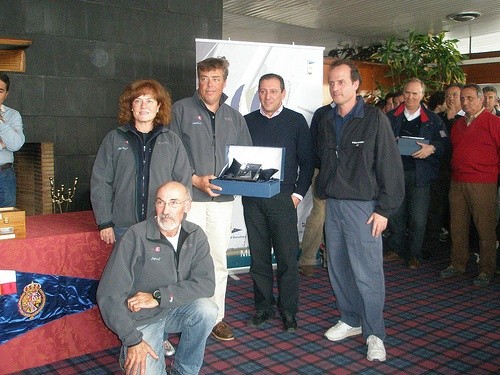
top-left (0, 211), bottom-right (126, 375)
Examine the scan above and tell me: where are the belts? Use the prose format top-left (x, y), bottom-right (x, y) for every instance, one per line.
top-left (0, 163), bottom-right (13, 170)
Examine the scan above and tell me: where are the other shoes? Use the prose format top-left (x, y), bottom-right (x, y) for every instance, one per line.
top-left (440, 265), bottom-right (462, 277)
top-left (299, 264), bottom-right (314, 277)
top-left (439, 227), bottom-right (448, 241)
top-left (383, 251), bottom-right (406, 262)
top-left (473, 271), bottom-right (493, 286)
top-left (163, 339), bottom-right (176, 355)
top-left (409, 256), bottom-right (420, 270)
top-left (119, 344), bottom-right (127, 372)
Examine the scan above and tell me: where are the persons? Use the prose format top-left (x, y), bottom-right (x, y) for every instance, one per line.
top-left (375, 77), bottom-right (500, 287)
top-left (316, 59), bottom-right (406, 364)
top-left (96, 181), bottom-right (219, 375)
top-left (89, 79), bottom-right (193, 357)
top-left (298, 102), bottom-right (338, 278)
top-left (241, 73), bottom-right (313, 334)
top-left (168, 57), bottom-right (253, 341)
top-left (0, 71), bottom-right (25, 207)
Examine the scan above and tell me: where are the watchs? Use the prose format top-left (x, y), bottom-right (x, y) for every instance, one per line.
top-left (153, 288), bottom-right (161, 305)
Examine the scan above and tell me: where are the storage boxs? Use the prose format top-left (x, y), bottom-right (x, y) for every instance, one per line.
top-left (0, 206), bottom-right (26, 239)
top-left (396, 138), bottom-right (429, 155)
top-left (210, 145), bottom-right (285, 198)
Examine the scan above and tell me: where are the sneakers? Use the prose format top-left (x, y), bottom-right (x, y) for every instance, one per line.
top-left (247, 309), bottom-right (275, 327)
top-left (324, 319), bottom-right (363, 341)
top-left (283, 312), bottom-right (297, 332)
top-left (366, 335), bottom-right (386, 362)
top-left (212, 321), bottom-right (235, 340)
